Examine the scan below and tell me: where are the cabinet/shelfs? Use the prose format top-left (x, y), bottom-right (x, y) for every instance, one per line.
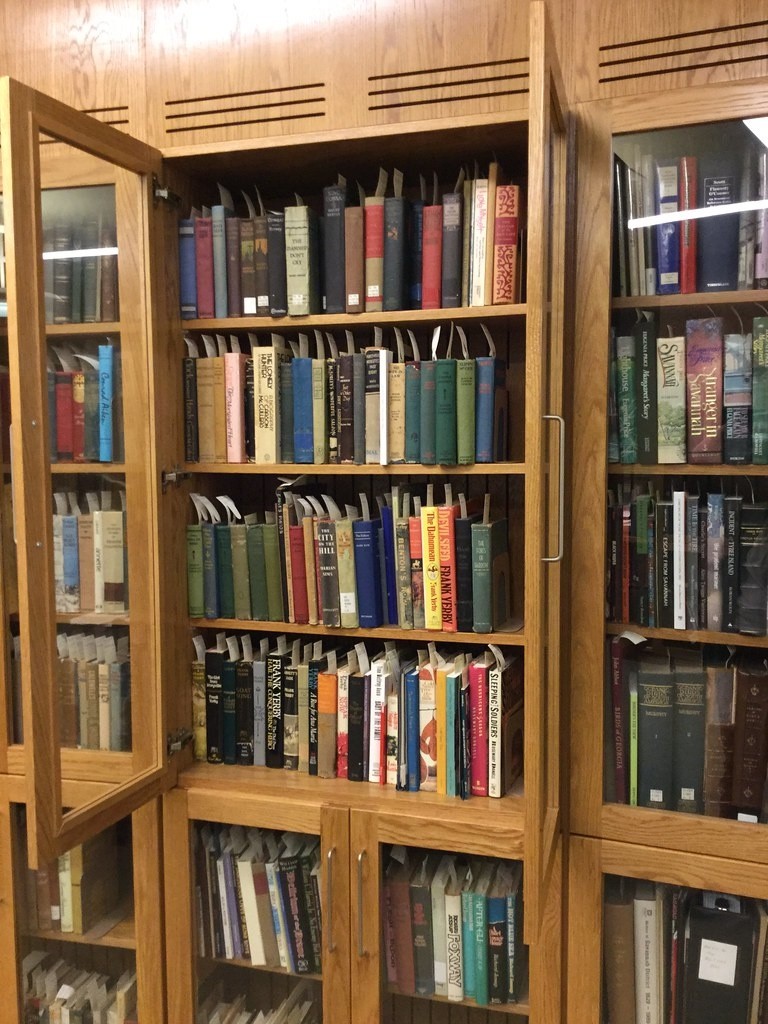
top-left (0, 0), bottom-right (768, 1024)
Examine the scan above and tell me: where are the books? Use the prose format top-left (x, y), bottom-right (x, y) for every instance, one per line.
top-left (185, 474), bottom-right (513, 634)
top-left (603, 881), bottom-right (768, 1024)
top-left (604, 490), bottom-right (768, 635)
top-left (604, 645), bottom-right (768, 818)
top-left (322, 179), bottom-right (523, 313)
top-left (18, 812), bottom-right (137, 1023)
top-left (183, 353), bottom-right (253, 461)
top-left (57, 655), bottom-right (131, 750)
top-left (53, 510), bottom-right (126, 613)
top-left (43, 207), bottom-right (118, 324)
top-left (380, 853), bottom-right (530, 1007)
top-left (193, 837), bottom-right (322, 1024)
top-left (615, 154), bottom-right (768, 295)
top-left (252, 344), bottom-right (512, 465)
top-left (190, 646), bottom-right (524, 800)
top-left (47, 345), bottom-right (124, 462)
top-left (178, 205), bottom-right (320, 320)
top-left (607, 315), bottom-right (768, 465)
